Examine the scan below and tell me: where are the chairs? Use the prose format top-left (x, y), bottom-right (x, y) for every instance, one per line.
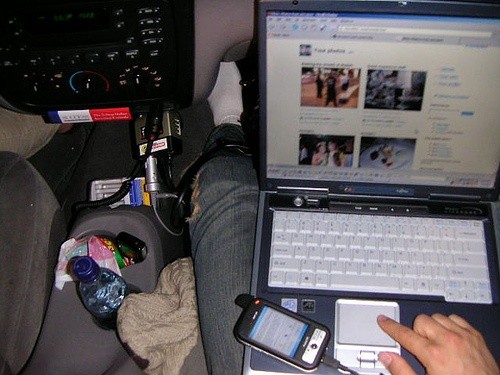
top-left (1, 148), bottom-right (68, 373)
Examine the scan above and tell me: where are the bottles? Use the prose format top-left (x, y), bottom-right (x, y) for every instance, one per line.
top-left (72, 253), bottom-right (127, 316)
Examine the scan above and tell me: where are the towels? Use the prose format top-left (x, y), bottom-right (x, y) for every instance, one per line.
top-left (114, 256), bottom-right (200, 375)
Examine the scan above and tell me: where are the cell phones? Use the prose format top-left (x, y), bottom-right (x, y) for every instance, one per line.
top-left (232, 293), bottom-right (333, 373)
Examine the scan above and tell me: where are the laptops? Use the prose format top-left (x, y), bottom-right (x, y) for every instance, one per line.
top-left (238, 0), bottom-right (500, 375)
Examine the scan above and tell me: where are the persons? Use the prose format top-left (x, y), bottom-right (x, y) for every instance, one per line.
top-left (312, 68), bottom-right (352, 106)
top-left (189, 60), bottom-right (500, 375)
top-left (312, 141), bottom-right (345, 167)
